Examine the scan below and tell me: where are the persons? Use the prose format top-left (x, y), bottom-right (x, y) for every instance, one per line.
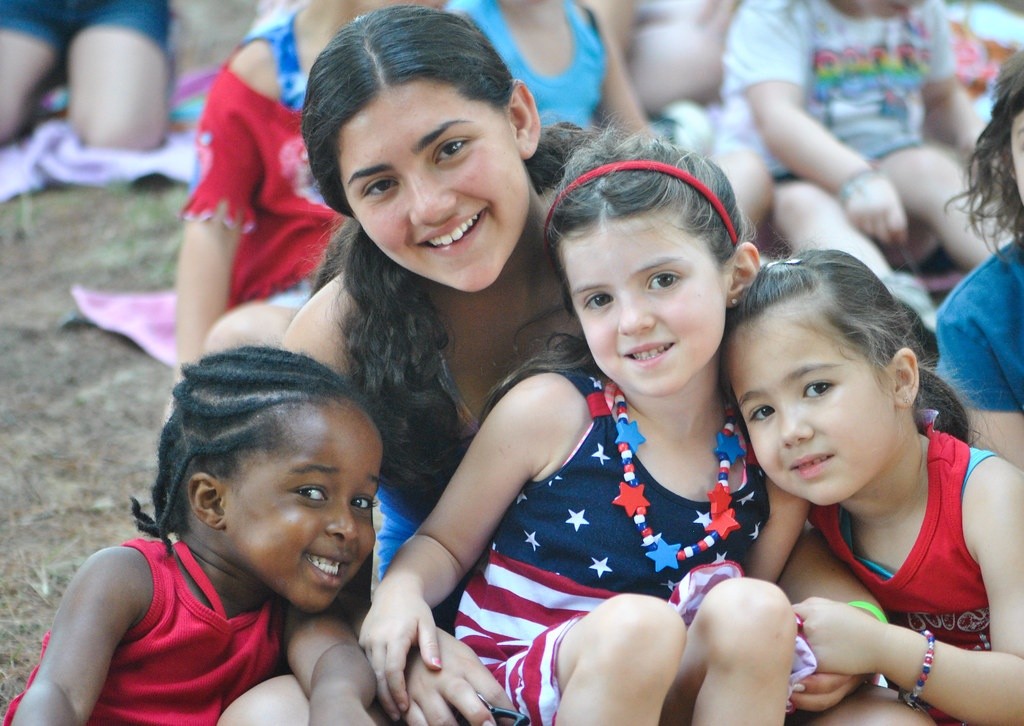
top-left (934, 50), bottom-right (1023, 470)
top-left (718, 247), bottom-right (1023, 726)
top-left (449, 2), bottom-right (807, 260)
top-left (720, 1), bottom-right (1017, 329)
top-left (0, 1), bottom-right (173, 154)
top-left (3, 343), bottom-right (385, 726)
top-left (358, 127), bottom-right (813, 726)
top-left (214, 4), bottom-right (940, 726)
top-left (178, 0), bottom-right (450, 370)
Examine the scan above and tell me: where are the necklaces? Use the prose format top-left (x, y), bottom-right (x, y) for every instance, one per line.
top-left (613, 385), bottom-right (747, 571)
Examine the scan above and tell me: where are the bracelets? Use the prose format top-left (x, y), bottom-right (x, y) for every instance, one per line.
top-left (899, 630), bottom-right (935, 710)
top-left (848, 600), bottom-right (888, 627)
top-left (840, 169), bottom-right (880, 198)
